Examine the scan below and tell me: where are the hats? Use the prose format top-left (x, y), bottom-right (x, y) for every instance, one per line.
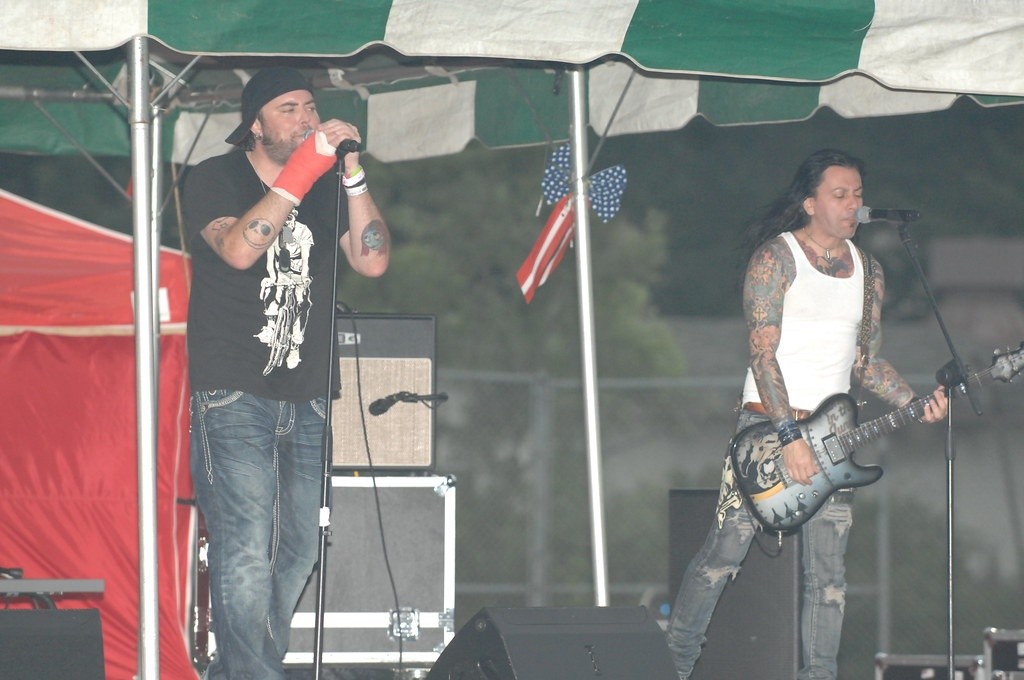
top-left (226, 67), bottom-right (313, 144)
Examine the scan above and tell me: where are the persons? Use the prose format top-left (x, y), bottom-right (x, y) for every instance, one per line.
top-left (663, 148), bottom-right (947, 678)
top-left (179, 65), bottom-right (392, 680)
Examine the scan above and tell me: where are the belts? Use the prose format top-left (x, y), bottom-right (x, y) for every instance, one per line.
top-left (743, 402), bottom-right (814, 421)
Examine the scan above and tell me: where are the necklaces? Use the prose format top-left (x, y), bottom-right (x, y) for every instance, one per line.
top-left (804, 226), bottom-right (845, 259)
top-left (250, 154), bottom-right (299, 243)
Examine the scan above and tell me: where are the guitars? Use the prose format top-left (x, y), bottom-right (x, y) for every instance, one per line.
top-left (730, 340), bottom-right (1024, 538)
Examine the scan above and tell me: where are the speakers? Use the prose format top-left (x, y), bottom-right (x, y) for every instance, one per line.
top-left (0, 608), bottom-right (106, 680)
top-left (425, 606), bottom-right (681, 680)
top-left (668, 489), bottom-right (805, 680)
top-left (329, 313), bottom-right (437, 471)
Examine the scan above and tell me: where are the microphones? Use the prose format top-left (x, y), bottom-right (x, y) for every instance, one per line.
top-left (852, 206), bottom-right (922, 224)
top-left (369, 391), bottom-right (411, 416)
top-left (304, 129), bottom-right (360, 153)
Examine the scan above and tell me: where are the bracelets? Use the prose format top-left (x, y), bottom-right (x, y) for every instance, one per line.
top-left (778, 419), bottom-right (801, 445)
top-left (340, 165), bottom-right (372, 197)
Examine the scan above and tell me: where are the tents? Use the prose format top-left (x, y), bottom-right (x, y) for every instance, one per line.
top-left (0, 191), bottom-right (200, 680)
top-left (0, 0), bottom-right (1024, 680)
